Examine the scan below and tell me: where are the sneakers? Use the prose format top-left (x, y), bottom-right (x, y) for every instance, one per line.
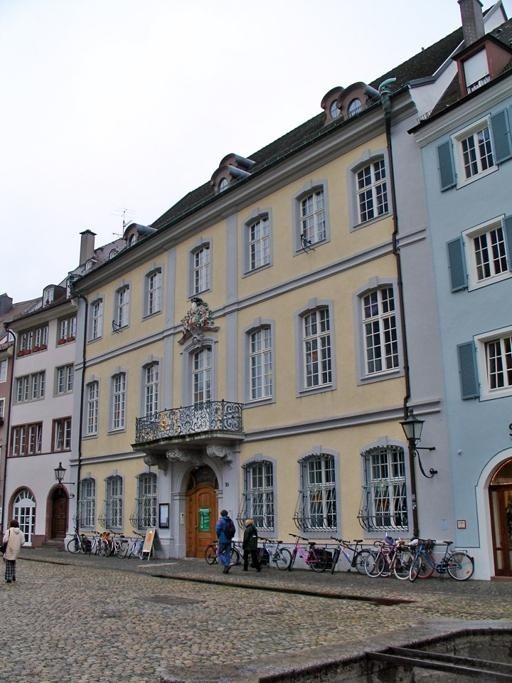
top-left (223, 565), bottom-right (231, 574)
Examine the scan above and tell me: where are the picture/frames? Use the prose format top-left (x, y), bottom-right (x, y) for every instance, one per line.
top-left (159, 503), bottom-right (169, 528)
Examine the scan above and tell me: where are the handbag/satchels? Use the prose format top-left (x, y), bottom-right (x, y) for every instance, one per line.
top-left (0, 541), bottom-right (8, 553)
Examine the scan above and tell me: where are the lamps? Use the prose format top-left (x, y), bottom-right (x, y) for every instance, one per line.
top-left (54, 462), bottom-right (75, 499)
top-left (399, 407), bottom-right (437, 478)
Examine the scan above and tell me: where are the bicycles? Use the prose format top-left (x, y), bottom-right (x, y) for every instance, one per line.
top-left (365, 537), bottom-right (437, 581)
top-left (250, 536), bottom-right (292, 570)
top-left (228, 538), bottom-right (261, 566)
top-left (408, 538), bottom-right (475, 582)
top-left (204, 539), bottom-right (240, 565)
top-left (287, 532), bottom-right (328, 573)
top-left (329, 534), bottom-right (376, 574)
top-left (66, 524), bottom-right (154, 561)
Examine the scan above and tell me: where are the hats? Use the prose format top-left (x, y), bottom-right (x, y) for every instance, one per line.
top-left (244, 518), bottom-right (254, 526)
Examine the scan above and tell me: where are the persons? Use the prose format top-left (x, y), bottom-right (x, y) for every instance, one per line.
top-left (216, 509), bottom-right (235, 572)
top-left (242, 518), bottom-right (262, 572)
top-left (1, 519), bottom-right (25, 583)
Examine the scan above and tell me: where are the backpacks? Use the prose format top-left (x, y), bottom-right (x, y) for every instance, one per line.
top-left (220, 517), bottom-right (235, 540)
